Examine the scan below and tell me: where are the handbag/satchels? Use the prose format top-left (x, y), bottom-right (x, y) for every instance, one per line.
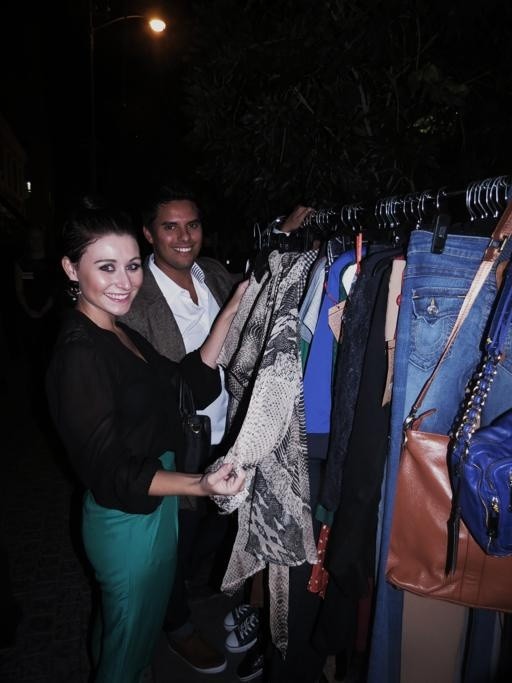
top-left (386, 413), bottom-right (512, 612)
top-left (176, 409), bottom-right (210, 473)
top-left (449, 377), bottom-right (510, 560)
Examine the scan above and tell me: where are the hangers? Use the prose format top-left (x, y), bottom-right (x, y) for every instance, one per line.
top-left (253, 175), bottom-right (512, 267)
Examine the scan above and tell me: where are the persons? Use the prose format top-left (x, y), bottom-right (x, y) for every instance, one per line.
top-left (122, 186), bottom-right (319, 676)
top-left (37, 201), bottom-right (257, 683)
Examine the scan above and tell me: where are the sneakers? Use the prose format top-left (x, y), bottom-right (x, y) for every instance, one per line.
top-left (224, 603), bottom-right (265, 681)
top-left (166, 629), bottom-right (228, 675)
top-left (191, 574), bottom-right (223, 605)
